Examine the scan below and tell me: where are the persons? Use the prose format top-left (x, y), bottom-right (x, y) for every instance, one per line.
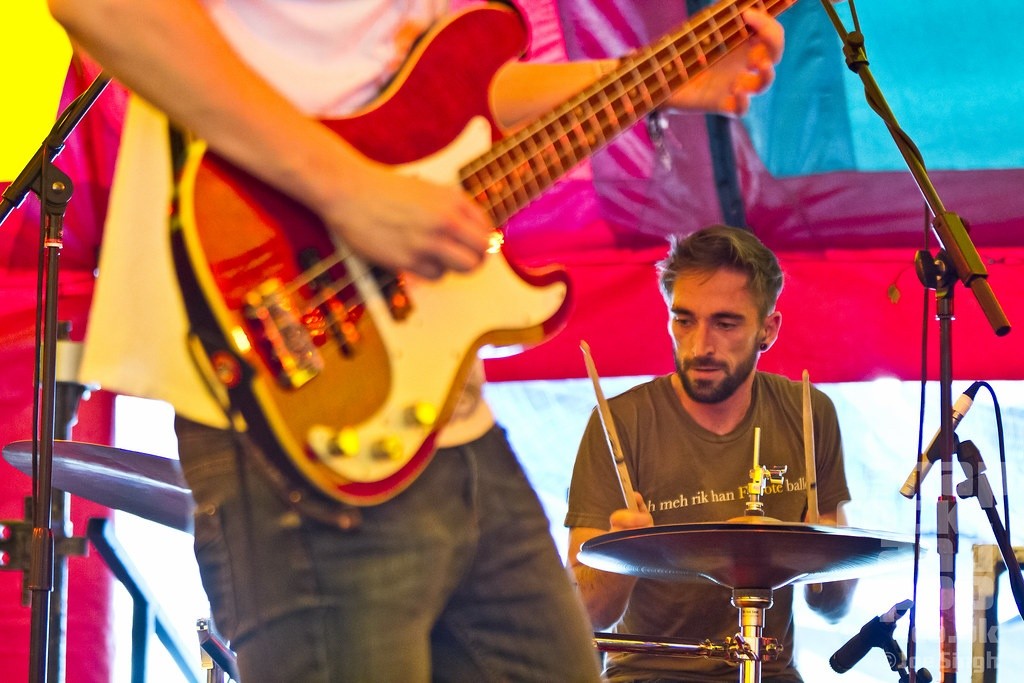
top-left (560, 223), bottom-right (857, 682)
top-left (42, 1), bottom-right (784, 682)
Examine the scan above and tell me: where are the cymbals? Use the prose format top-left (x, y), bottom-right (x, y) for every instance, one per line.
top-left (573, 513), bottom-right (929, 588)
top-left (1, 437), bottom-right (194, 535)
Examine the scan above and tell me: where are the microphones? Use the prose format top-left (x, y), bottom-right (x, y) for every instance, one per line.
top-left (831, 599), bottom-right (912, 673)
top-left (900, 381), bottom-right (982, 499)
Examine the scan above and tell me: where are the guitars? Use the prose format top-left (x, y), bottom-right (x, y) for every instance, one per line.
top-left (168, 0), bottom-right (798, 528)
